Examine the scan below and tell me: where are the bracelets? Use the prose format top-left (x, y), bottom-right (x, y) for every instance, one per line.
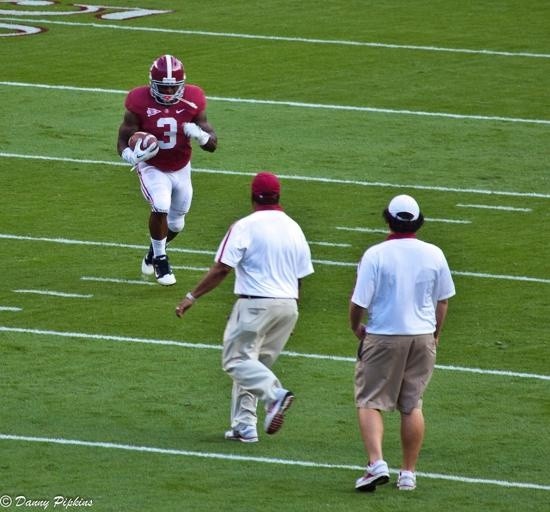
top-left (185, 292), bottom-right (196, 301)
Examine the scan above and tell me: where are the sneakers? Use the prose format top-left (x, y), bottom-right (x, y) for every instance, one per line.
top-left (141, 254), bottom-right (155, 276)
top-left (355, 460), bottom-right (390, 492)
top-left (224, 429), bottom-right (259, 442)
top-left (263, 392), bottom-right (294, 434)
top-left (151, 254), bottom-right (176, 286)
top-left (396, 470), bottom-right (417, 491)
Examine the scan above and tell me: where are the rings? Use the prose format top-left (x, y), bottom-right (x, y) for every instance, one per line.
top-left (175, 307), bottom-right (180, 310)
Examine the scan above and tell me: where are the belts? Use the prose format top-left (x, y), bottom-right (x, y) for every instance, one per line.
top-left (238, 295), bottom-right (266, 299)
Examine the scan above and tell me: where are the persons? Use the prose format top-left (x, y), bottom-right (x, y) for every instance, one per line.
top-left (347, 194), bottom-right (457, 492)
top-left (115, 54), bottom-right (218, 287)
top-left (174, 169), bottom-right (315, 443)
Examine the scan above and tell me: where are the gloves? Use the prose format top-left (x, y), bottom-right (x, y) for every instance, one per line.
top-left (121, 137), bottom-right (160, 166)
top-left (182, 122), bottom-right (210, 146)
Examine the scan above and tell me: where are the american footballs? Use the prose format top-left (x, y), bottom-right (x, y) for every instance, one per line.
top-left (128, 131), bottom-right (158, 151)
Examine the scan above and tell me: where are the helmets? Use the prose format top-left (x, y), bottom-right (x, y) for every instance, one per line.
top-left (148, 54), bottom-right (187, 106)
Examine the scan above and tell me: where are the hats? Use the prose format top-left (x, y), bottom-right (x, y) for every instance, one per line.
top-left (251, 171), bottom-right (281, 204)
top-left (388, 194), bottom-right (420, 221)
